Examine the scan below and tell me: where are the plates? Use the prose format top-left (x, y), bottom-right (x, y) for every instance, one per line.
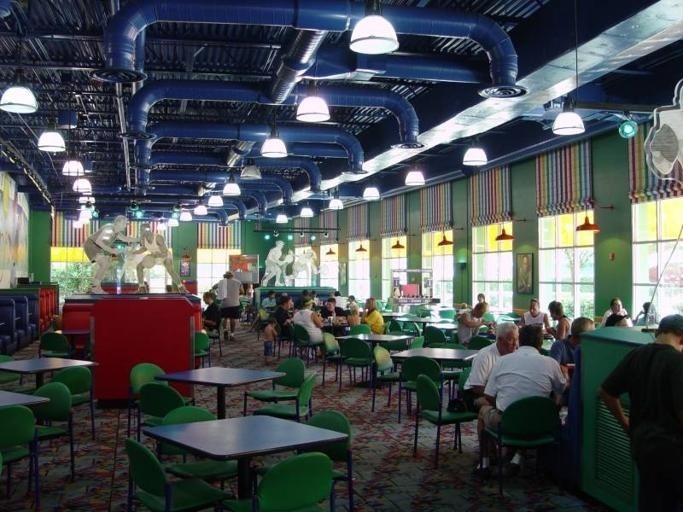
top-left (333, 321), bottom-right (347, 324)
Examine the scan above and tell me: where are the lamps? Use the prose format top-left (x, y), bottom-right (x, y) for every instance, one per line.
top-left (551, 92), bottom-right (585, 135)
top-left (575, 205), bottom-right (601, 233)
top-left (439, 232), bottom-right (454, 247)
top-left (239, 156), bottom-right (263, 181)
top-left (60, 148), bottom-right (86, 177)
top-left (615, 117), bottom-right (637, 139)
top-left (0, 64), bottom-right (40, 117)
top-left (404, 160), bottom-right (426, 187)
top-left (496, 225), bottom-right (513, 241)
top-left (391, 238), bottom-right (405, 249)
top-left (462, 137), bottom-right (489, 168)
top-left (260, 127), bottom-right (289, 159)
top-left (295, 79), bottom-right (331, 124)
top-left (58, 179), bottom-right (388, 262)
top-left (348, 1), bottom-right (400, 56)
top-left (37, 117), bottom-right (67, 154)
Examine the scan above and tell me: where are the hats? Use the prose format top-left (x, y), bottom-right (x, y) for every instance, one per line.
top-left (301, 297), bottom-right (316, 306)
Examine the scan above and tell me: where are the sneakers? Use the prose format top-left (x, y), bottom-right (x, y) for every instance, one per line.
top-left (471, 461), bottom-right (521, 480)
top-left (224, 330), bottom-right (229, 340)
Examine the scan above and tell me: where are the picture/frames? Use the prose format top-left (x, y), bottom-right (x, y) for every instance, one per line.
top-left (516, 252), bottom-right (534, 295)
top-left (179, 261), bottom-right (191, 278)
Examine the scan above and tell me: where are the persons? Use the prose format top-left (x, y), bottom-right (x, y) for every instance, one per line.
top-left (551, 316), bottom-right (595, 365)
top-left (602, 314), bottom-right (683, 512)
top-left (3, 186), bottom-right (27, 270)
top-left (129, 224), bottom-right (189, 294)
top-left (458, 295), bottom-right (496, 345)
top-left (468, 322), bottom-right (570, 477)
top-left (520, 298), bottom-right (572, 337)
top-left (599, 298), bottom-right (658, 328)
top-left (200, 271), bottom-right (384, 347)
top-left (84, 214), bottom-right (141, 295)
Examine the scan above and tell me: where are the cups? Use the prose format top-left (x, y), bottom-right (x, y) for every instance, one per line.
top-left (327, 315), bottom-right (333, 325)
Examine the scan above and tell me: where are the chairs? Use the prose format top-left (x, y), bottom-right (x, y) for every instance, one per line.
top-left (234, 287), bottom-right (659, 509)
top-left (2, 287), bottom-right (361, 511)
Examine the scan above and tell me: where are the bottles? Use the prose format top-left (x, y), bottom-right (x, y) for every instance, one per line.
top-left (487, 324), bottom-right (495, 335)
